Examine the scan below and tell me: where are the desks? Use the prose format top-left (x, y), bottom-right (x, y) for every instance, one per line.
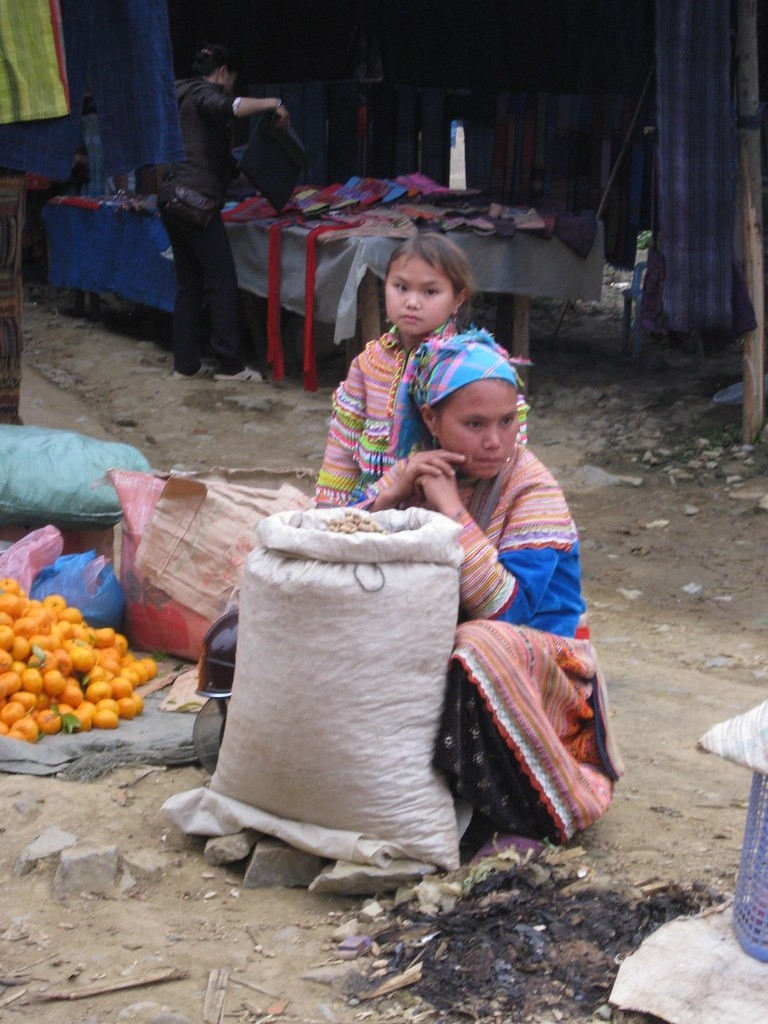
top-left (43, 196), bottom-right (605, 399)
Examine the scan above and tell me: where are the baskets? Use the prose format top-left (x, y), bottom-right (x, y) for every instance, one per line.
top-left (733, 769), bottom-right (768, 962)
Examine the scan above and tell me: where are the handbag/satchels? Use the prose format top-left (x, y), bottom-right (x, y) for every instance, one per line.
top-left (29, 550), bottom-right (124, 634)
top-left (165, 184), bottom-right (220, 227)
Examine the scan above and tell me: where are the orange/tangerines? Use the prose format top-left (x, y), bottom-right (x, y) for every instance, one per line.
top-left (0, 576), bottom-right (157, 745)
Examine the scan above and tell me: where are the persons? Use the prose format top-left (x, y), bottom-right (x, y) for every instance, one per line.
top-left (156, 41), bottom-right (293, 383)
top-left (346, 326), bottom-right (625, 840)
top-left (314, 231), bottom-right (529, 509)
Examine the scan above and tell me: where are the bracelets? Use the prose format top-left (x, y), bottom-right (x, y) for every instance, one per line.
top-left (273, 98), bottom-right (282, 111)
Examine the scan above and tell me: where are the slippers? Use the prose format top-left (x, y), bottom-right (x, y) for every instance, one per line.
top-left (213, 366), bottom-right (268, 383)
top-left (173, 363), bottom-right (216, 380)
top-left (467, 832), bottom-right (548, 866)
